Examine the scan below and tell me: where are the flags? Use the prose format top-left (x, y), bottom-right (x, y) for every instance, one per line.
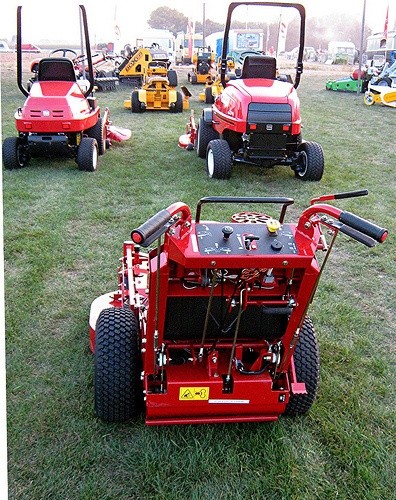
top-left (278, 16), bottom-right (294, 53)
top-left (383, 9), bottom-right (389, 38)
top-left (188, 17), bottom-right (195, 37)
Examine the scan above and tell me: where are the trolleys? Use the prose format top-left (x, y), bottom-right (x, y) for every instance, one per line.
top-left (178, 0), bottom-right (327, 186)
top-left (2, 3), bottom-right (132, 170)
top-left (91, 190), bottom-right (389, 429)
top-left (2, 24), bottom-right (243, 114)
